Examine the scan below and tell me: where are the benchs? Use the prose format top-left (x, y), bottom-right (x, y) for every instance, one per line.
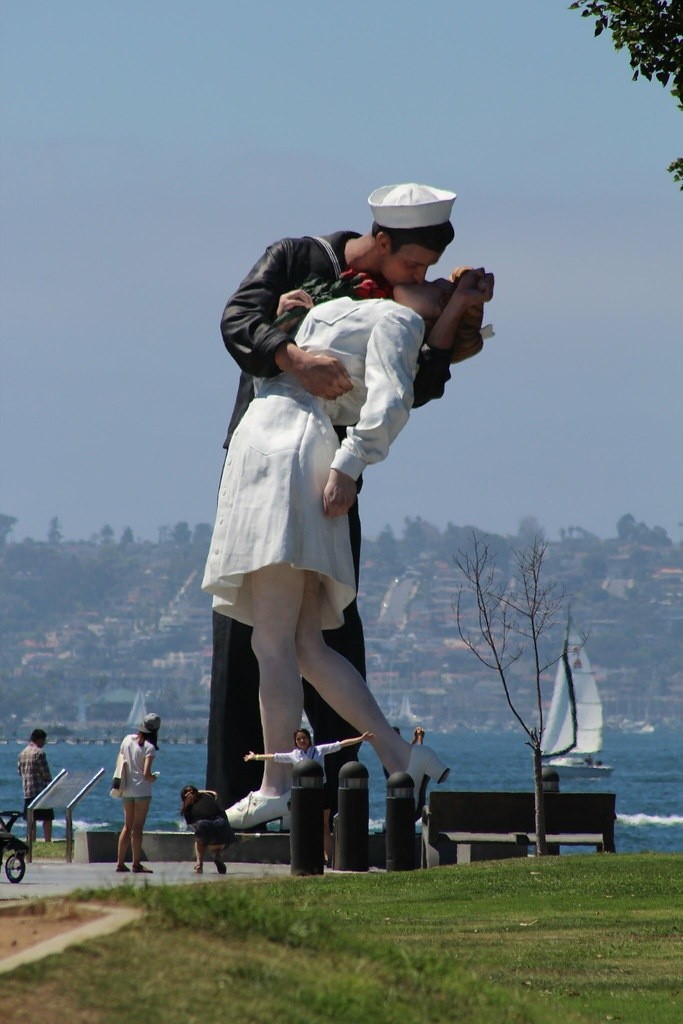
top-left (421, 792), bottom-right (617, 870)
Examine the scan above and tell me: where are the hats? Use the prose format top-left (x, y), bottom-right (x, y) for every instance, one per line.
top-left (139, 712), bottom-right (161, 733)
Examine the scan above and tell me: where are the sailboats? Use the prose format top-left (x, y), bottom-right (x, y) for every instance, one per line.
top-left (534, 607), bottom-right (616, 778)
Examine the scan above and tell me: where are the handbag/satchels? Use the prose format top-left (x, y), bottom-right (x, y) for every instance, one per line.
top-left (109, 753), bottom-right (124, 799)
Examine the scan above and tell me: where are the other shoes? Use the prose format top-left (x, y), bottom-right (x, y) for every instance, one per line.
top-left (116, 864), bottom-right (130, 872)
top-left (132, 864), bottom-right (153, 873)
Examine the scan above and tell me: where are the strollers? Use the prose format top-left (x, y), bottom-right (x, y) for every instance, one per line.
top-left (0, 812), bottom-right (30, 883)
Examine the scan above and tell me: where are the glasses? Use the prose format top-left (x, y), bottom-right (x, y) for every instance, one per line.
top-left (43, 739), bottom-right (47, 743)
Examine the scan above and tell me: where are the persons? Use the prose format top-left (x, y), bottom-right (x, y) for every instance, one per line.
top-left (17, 726), bottom-right (55, 843)
top-left (110, 713), bottom-right (161, 874)
top-left (243, 727), bottom-right (376, 867)
top-left (383, 726), bottom-right (424, 779)
top-left (200, 183), bottom-right (495, 830)
top-left (180, 785), bottom-right (235, 874)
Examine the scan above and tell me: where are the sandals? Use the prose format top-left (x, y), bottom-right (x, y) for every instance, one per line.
top-left (193, 863), bottom-right (203, 873)
top-left (217, 861), bottom-right (226, 873)
